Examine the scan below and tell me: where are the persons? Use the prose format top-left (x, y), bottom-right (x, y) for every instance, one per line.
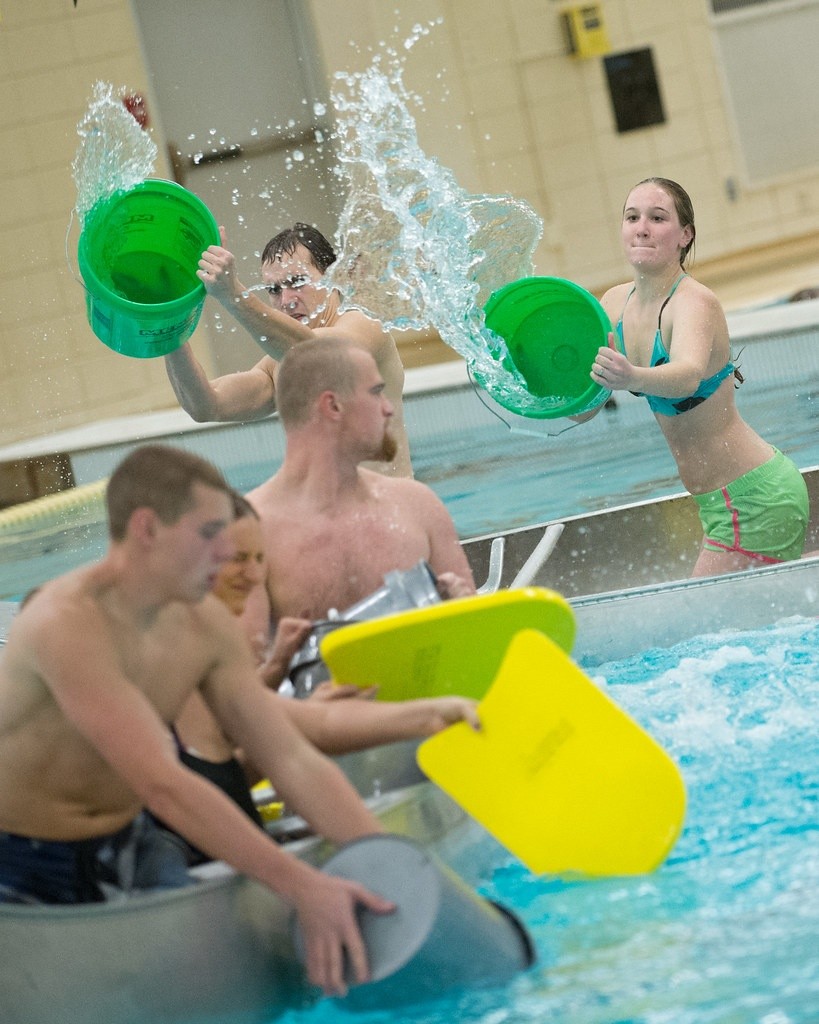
top-left (0, 340), bottom-right (482, 1000)
top-left (563, 178), bottom-right (809, 577)
top-left (113, 222), bottom-right (414, 482)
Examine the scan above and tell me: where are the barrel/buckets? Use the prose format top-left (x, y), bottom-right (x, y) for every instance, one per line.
top-left (316, 559), bottom-right (443, 623)
top-left (65, 179), bottom-right (220, 359)
top-left (296, 618), bottom-right (444, 799)
top-left (304, 835), bottom-right (536, 1014)
top-left (464, 278), bottom-right (610, 437)
